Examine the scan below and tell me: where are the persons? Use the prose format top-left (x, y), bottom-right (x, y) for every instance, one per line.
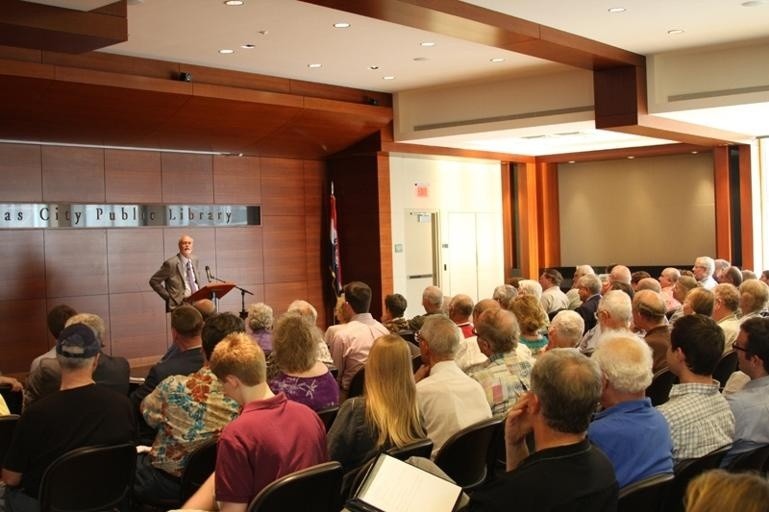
top-left (406, 285), bottom-right (444, 332)
top-left (246, 301), bottom-right (273, 351)
top-left (403, 347), bottom-right (619, 512)
top-left (172, 331), bottom-right (329, 512)
top-left (741, 270), bottom-right (757, 282)
top-left (456, 299), bottom-right (532, 372)
top-left (492, 284), bottom-right (518, 310)
top-left (637, 277), bottom-right (661, 294)
top-left (668, 275), bottom-right (698, 326)
top-left (128, 303), bottom-right (207, 442)
top-left (25, 312), bottom-right (130, 409)
top-left (710, 283), bottom-right (740, 357)
top-left (330, 281), bottom-right (391, 405)
top-left (415, 313), bottom-right (493, 463)
top-left (539, 309), bottom-right (585, 355)
top-left (759, 270), bottom-right (769, 284)
top-left (719, 316), bottom-right (769, 469)
top-left (566, 264), bottom-right (595, 310)
top-left (692, 256), bottom-right (719, 292)
top-left (658, 268), bottom-right (683, 315)
top-left (324, 293), bottom-right (352, 356)
top-left (631, 271), bottom-right (650, 291)
top-left (133, 310), bottom-right (245, 512)
top-left (325, 334), bottom-right (427, 474)
top-left (0, 321), bottom-right (135, 511)
top-left (193, 297), bottom-right (217, 322)
top-left (738, 279), bottom-right (769, 329)
top-left (447, 294), bottom-right (478, 339)
top-left (601, 264), bottom-right (631, 294)
top-left (682, 468), bottom-right (769, 512)
top-left (585, 328), bottom-right (675, 490)
top-left (148, 234), bottom-right (201, 312)
top-left (581, 289), bottom-right (632, 357)
top-left (717, 266), bottom-right (744, 287)
top-left (381, 293), bottom-right (420, 336)
top-left (506, 293), bottom-right (550, 358)
top-left (574, 273), bottom-right (602, 335)
top-left (267, 299), bottom-right (333, 364)
top-left (541, 268), bottom-right (570, 319)
top-left (682, 286), bottom-right (714, 315)
top-left (268, 312), bottom-right (339, 412)
top-left (653, 314), bottom-right (735, 469)
top-left (517, 279), bottom-right (543, 302)
top-left (631, 288), bottom-right (672, 374)
top-left (713, 258), bottom-right (729, 283)
top-left (606, 282), bottom-right (634, 298)
top-left (0, 375), bottom-right (23, 418)
top-left (30, 304), bottom-right (78, 372)
top-left (465, 307), bottom-right (537, 422)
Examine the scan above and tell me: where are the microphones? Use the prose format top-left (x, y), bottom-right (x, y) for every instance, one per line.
top-left (205, 266), bottom-right (212, 283)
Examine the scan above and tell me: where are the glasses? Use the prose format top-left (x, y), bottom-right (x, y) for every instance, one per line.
top-left (471, 326), bottom-right (482, 338)
top-left (594, 309), bottom-right (608, 319)
top-left (732, 340), bottom-right (749, 354)
top-left (414, 330), bottom-right (426, 344)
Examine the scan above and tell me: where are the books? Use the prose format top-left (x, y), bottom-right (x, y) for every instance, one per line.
top-left (340, 449), bottom-right (464, 512)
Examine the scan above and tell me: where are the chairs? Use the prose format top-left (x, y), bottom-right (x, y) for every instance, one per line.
top-left (396, 330), bottom-right (419, 346)
top-left (347, 365), bottom-right (365, 398)
top-left (178, 436), bottom-right (216, 505)
top-left (646, 370), bottom-right (680, 408)
top-left (33, 441), bottom-right (139, 511)
top-left (317, 408), bottom-right (344, 431)
top-left (436, 418), bottom-right (508, 492)
top-left (713, 350), bottom-right (741, 387)
top-left (677, 439), bottom-right (732, 486)
top-left (723, 441), bottom-right (767, 471)
top-left (412, 353), bottom-right (424, 374)
top-left (349, 440), bottom-right (433, 506)
top-left (0, 415), bottom-right (18, 451)
top-left (618, 473), bottom-right (677, 511)
top-left (248, 458), bottom-right (343, 510)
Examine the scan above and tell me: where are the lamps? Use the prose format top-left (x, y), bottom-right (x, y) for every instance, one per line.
top-left (335, 24), bottom-right (350, 28)
top-left (226, 1), bottom-right (244, 6)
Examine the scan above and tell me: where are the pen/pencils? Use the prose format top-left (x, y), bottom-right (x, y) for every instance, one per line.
top-left (519, 379), bottom-right (527, 391)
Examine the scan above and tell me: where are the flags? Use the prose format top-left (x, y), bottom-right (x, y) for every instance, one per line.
top-left (327, 194), bottom-right (343, 298)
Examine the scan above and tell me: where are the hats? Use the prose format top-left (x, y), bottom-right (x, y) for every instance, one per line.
top-left (56, 322), bottom-right (103, 358)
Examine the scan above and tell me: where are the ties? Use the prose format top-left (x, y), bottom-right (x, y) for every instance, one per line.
top-left (186, 262), bottom-right (197, 295)
